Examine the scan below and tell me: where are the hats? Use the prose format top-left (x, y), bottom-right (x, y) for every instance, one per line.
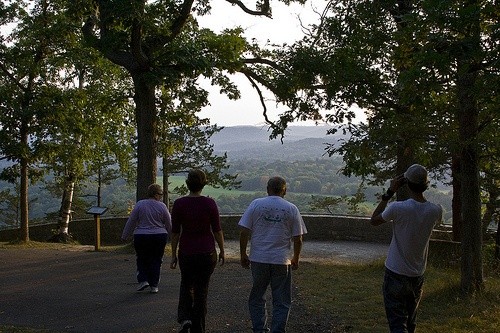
top-left (404, 164), bottom-right (427, 184)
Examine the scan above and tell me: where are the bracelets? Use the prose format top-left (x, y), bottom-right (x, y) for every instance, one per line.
top-left (387, 188), bottom-right (395, 198)
top-left (381, 194), bottom-right (390, 201)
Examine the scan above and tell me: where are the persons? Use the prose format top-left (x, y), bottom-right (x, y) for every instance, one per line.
top-left (371, 164), bottom-right (442, 333)
top-left (121, 184), bottom-right (172, 294)
top-left (170, 170), bottom-right (225, 333)
top-left (236, 176), bottom-right (309, 332)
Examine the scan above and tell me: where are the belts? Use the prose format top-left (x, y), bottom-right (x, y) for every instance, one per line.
top-left (385, 270), bottom-right (422, 280)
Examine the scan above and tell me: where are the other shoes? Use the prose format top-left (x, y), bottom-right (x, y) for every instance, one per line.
top-left (177, 320), bottom-right (192, 333)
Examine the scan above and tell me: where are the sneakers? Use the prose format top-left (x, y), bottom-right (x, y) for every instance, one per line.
top-left (151, 287), bottom-right (159, 292)
top-left (136, 281), bottom-right (149, 291)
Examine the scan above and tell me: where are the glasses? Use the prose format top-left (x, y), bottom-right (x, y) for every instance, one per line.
top-left (157, 192), bottom-right (163, 196)
